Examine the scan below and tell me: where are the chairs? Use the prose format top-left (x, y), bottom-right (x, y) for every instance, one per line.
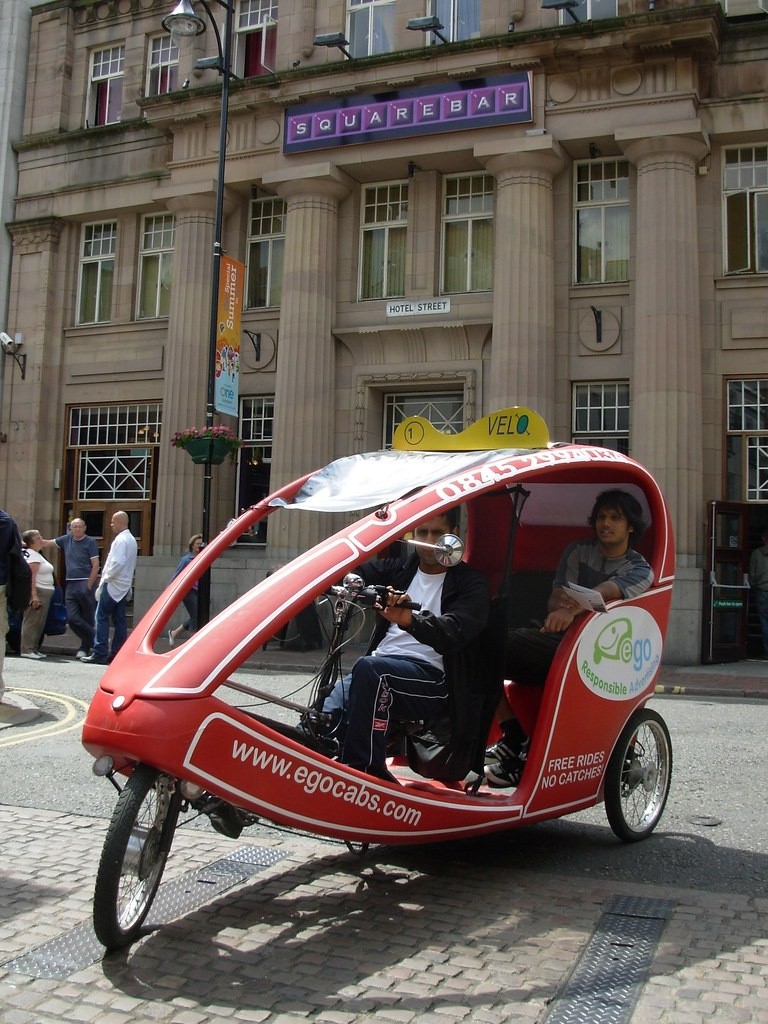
top-left (494, 570), bottom-right (553, 630)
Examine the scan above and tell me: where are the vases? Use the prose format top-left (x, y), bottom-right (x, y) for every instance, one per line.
top-left (185, 439), bottom-right (230, 464)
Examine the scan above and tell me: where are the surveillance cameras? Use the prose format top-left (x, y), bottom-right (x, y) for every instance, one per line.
top-left (0, 331), bottom-right (14, 347)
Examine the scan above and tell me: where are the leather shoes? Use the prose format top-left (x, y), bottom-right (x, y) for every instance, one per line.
top-left (107, 656), bottom-right (113, 663)
top-left (81, 655), bottom-right (107, 664)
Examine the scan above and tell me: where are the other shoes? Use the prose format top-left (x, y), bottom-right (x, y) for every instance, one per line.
top-left (21, 652), bottom-right (41, 659)
top-left (75, 651), bottom-right (86, 658)
top-left (191, 791), bottom-right (244, 841)
top-left (37, 651), bottom-right (47, 658)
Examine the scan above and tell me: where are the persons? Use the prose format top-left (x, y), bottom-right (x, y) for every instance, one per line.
top-left (81, 511), bottom-right (138, 664)
top-left (20, 518), bottom-right (100, 659)
top-left (748, 527), bottom-right (768, 653)
top-left (0, 509), bottom-right (22, 704)
top-left (189, 488), bottom-right (655, 839)
top-left (168, 534), bottom-right (202, 645)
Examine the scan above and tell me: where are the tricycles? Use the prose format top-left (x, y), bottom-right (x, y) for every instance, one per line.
top-left (80, 404), bottom-right (675, 952)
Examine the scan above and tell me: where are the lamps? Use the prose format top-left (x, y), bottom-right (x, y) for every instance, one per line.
top-left (313, 32), bottom-right (355, 62)
top-left (407, 16), bottom-right (448, 42)
top-left (542, 0), bottom-right (579, 23)
top-left (195, 55), bottom-right (244, 82)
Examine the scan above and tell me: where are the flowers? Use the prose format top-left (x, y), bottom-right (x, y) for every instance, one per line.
top-left (176, 425), bottom-right (240, 448)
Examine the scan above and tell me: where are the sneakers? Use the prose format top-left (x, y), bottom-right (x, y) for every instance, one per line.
top-left (484, 736), bottom-right (530, 765)
top-left (483, 749), bottom-right (528, 787)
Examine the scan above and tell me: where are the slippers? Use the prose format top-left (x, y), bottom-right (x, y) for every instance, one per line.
top-left (168, 630), bottom-right (176, 649)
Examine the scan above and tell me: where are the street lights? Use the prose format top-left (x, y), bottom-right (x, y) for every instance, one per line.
top-left (157, 0), bottom-right (238, 630)
